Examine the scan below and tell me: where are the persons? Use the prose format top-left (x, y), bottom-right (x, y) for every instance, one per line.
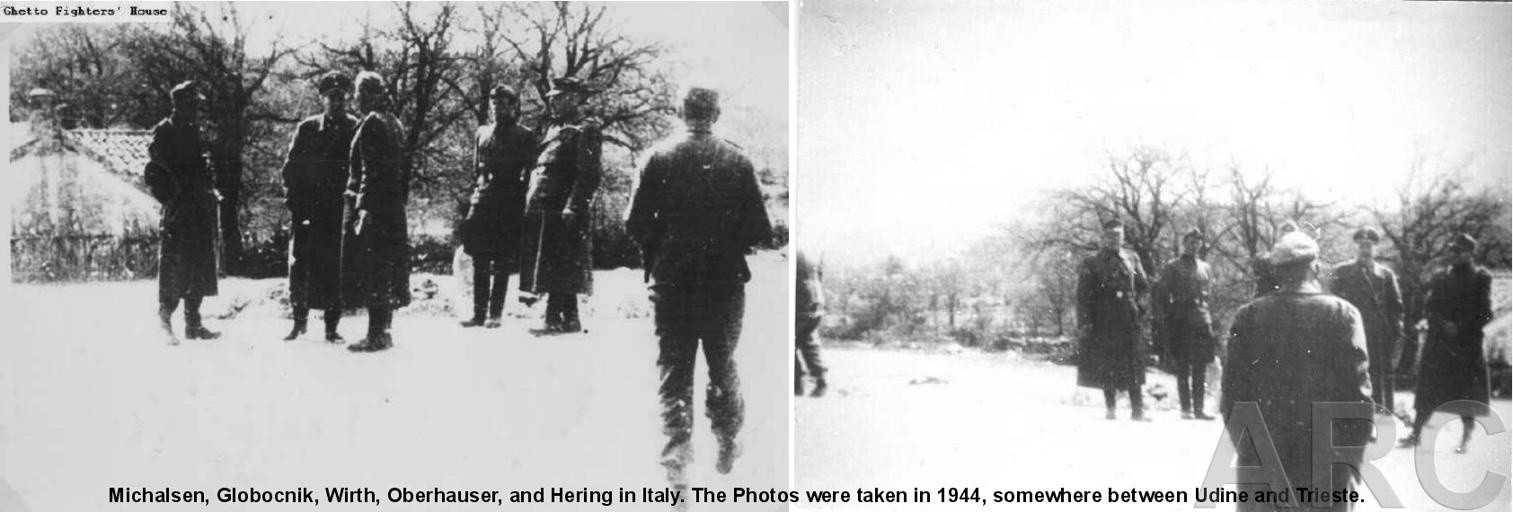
top-left (1250, 220), bottom-right (1298, 300)
top-left (1396, 231), bottom-right (1493, 452)
top-left (1328, 228), bottom-right (1405, 442)
top-left (623, 83), bottom-right (774, 479)
top-left (1074, 218), bottom-right (1150, 422)
top-left (794, 247), bottom-right (829, 398)
top-left (1153, 227), bottom-right (1215, 423)
top-left (1218, 230), bottom-right (1377, 511)
top-left (143, 79), bottom-right (221, 345)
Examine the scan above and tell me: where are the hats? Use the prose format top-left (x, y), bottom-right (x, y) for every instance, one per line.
top-left (1270, 231), bottom-right (1321, 265)
top-left (544, 75), bottom-right (581, 98)
top-left (684, 85), bottom-right (721, 111)
top-left (318, 72), bottom-right (351, 98)
top-left (1182, 226), bottom-right (1205, 242)
top-left (488, 84), bottom-right (519, 102)
top-left (169, 80), bottom-right (208, 102)
top-left (1445, 230), bottom-right (1479, 256)
top-left (1352, 227), bottom-right (1380, 246)
top-left (1102, 218), bottom-right (1126, 234)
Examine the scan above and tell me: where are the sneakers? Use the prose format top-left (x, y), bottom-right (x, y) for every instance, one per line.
top-left (283, 320), bottom-right (307, 342)
top-left (1398, 431), bottom-right (1424, 447)
top-left (715, 435), bottom-right (739, 475)
top-left (346, 332), bottom-right (393, 353)
top-left (1455, 439), bottom-right (1473, 455)
top-left (794, 376), bottom-right (828, 397)
top-left (536, 319), bottom-right (581, 335)
top-left (460, 314), bottom-right (504, 328)
top-left (1103, 403), bottom-right (1152, 422)
top-left (325, 328), bottom-right (347, 344)
top-left (162, 331), bottom-right (181, 346)
top-left (184, 326), bottom-right (222, 340)
top-left (1180, 408), bottom-right (1216, 421)
